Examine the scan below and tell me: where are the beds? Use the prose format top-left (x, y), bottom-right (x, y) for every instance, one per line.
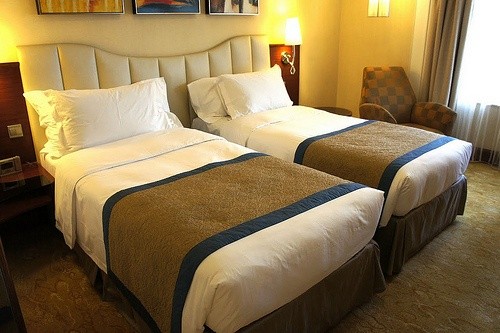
top-left (15, 36), bottom-right (472, 333)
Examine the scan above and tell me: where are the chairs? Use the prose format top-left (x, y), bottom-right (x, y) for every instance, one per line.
top-left (359, 66), bottom-right (458, 137)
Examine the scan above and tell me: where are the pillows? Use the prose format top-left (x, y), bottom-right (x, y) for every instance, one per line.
top-left (187, 77), bottom-right (229, 123)
top-left (214, 64), bottom-right (293, 120)
top-left (23, 91), bottom-right (68, 159)
top-left (43, 77), bottom-right (184, 156)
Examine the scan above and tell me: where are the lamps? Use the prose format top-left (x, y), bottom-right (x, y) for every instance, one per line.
top-left (281, 18), bottom-right (302, 75)
top-left (367, 0), bottom-right (390, 17)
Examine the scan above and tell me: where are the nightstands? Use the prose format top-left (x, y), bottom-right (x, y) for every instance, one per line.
top-left (0, 161), bottom-right (55, 252)
top-left (313, 106), bottom-right (352, 117)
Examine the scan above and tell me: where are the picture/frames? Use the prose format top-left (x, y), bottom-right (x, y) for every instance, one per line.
top-left (205, 0), bottom-right (259, 15)
top-left (35, 0), bottom-right (125, 15)
top-left (132, 0), bottom-right (201, 15)
